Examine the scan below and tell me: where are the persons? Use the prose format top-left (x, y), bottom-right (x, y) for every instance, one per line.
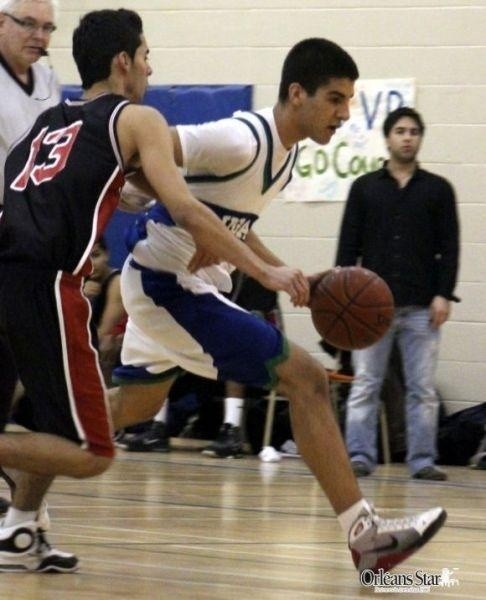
top-left (336, 109), bottom-right (466, 482)
top-left (0, 1), bottom-right (451, 590)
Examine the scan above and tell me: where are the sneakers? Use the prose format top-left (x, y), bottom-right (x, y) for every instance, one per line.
top-left (412, 466), bottom-right (447, 480)
top-left (0, 465), bottom-right (49, 532)
top-left (117, 422), bottom-right (170, 453)
top-left (200, 423), bottom-right (246, 460)
top-left (0, 516), bottom-right (81, 574)
top-left (350, 461), bottom-right (370, 477)
top-left (348, 500), bottom-right (446, 585)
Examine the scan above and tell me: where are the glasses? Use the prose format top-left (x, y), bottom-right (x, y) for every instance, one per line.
top-left (2, 12), bottom-right (56, 35)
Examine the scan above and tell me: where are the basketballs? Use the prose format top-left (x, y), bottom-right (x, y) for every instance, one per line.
top-left (309, 268), bottom-right (394, 352)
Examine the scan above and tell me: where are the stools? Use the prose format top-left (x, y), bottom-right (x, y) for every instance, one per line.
top-left (258, 369), bottom-right (390, 471)
